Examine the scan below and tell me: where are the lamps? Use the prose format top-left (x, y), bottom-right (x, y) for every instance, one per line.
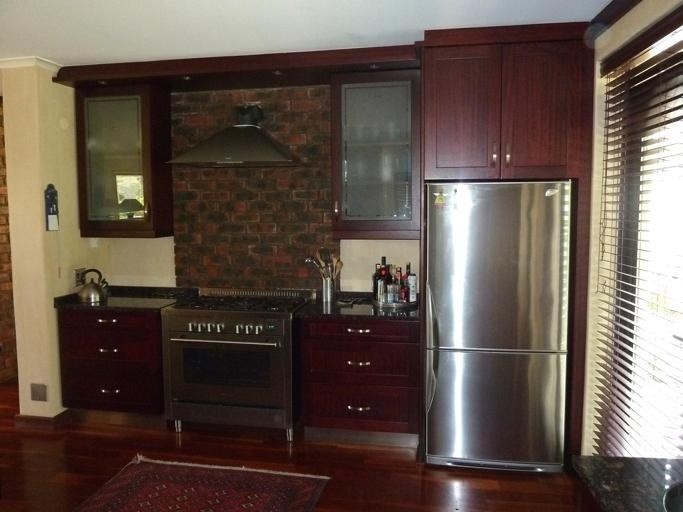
top-left (119, 199), bottom-right (144, 218)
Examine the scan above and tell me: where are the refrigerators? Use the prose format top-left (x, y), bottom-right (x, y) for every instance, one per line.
top-left (424, 179), bottom-right (573, 475)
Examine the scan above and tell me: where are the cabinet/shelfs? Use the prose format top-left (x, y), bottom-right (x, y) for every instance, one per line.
top-left (299, 322), bottom-right (420, 434)
top-left (58, 313), bottom-right (164, 415)
top-left (330, 69), bottom-right (421, 239)
top-left (420, 41), bottom-right (594, 180)
top-left (76, 86), bottom-right (174, 237)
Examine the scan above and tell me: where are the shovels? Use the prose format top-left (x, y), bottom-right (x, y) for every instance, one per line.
top-left (319, 248), bottom-right (330, 269)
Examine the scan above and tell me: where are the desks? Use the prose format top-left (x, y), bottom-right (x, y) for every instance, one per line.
top-left (572, 455), bottom-right (683, 512)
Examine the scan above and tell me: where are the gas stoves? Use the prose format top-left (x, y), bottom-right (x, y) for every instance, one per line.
top-left (171, 291), bottom-right (304, 314)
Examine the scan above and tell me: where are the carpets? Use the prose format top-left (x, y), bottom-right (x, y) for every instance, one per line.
top-left (71, 452), bottom-right (331, 512)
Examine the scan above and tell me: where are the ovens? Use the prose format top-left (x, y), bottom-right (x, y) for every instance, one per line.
top-left (160, 315), bottom-right (293, 431)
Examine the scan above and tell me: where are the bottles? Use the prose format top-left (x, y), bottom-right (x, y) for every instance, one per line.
top-left (372, 256), bottom-right (418, 304)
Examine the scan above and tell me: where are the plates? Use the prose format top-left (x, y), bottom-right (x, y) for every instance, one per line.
top-left (372, 297), bottom-right (419, 308)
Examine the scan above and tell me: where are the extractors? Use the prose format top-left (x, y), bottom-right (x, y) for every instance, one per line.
top-left (162, 102), bottom-right (305, 169)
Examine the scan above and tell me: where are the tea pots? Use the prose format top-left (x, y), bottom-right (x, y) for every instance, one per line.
top-left (75, 269), bottom-right (111, 303)
